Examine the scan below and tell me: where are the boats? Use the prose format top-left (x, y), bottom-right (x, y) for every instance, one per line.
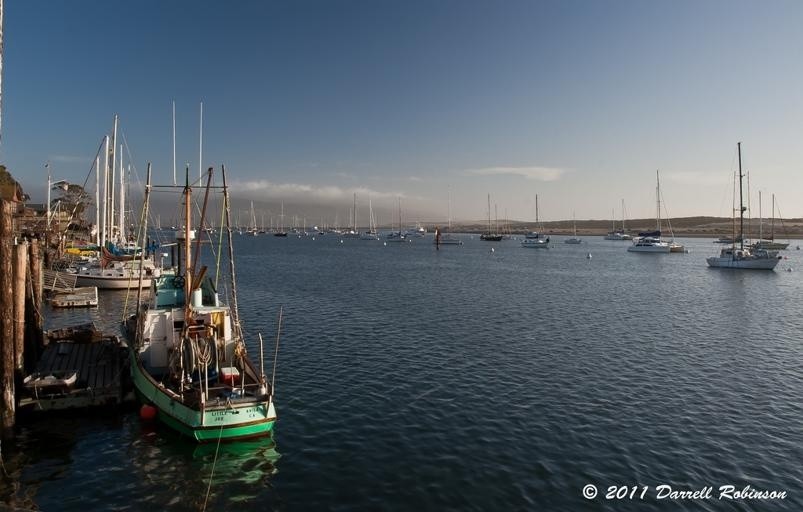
top-left (54, 112), bottom-right (179, 288)
top-left (122, 160), bottom-right (277, 442)
